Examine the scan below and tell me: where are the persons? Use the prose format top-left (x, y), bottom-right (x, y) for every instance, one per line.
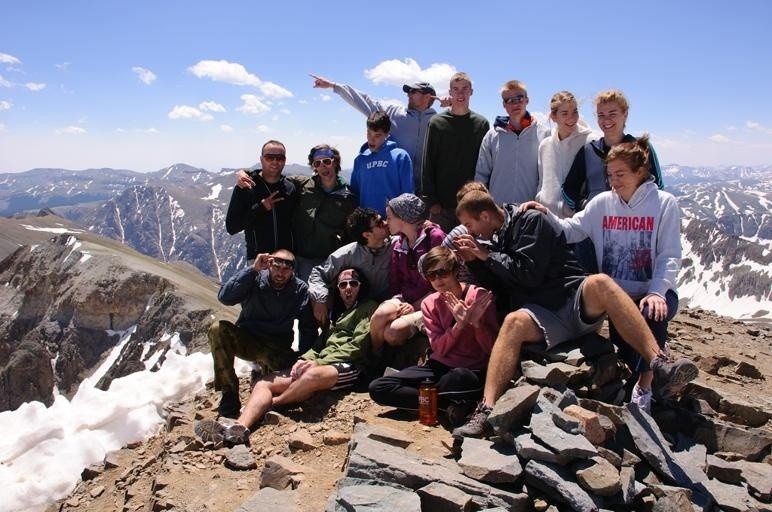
top-left (529, 90), bottom-right (589, 218)
top-left (199, 178), bottom-right (704, 443)
top-left (222, 141), bottom-right (297, 263)
top-left (307, 73), bottom-right (436, 197)
top-left (346, 112), bottom-right (417, 213)
top-left (563, 90), bottom-right (666, 218)
top-left (419, 72), bottom-right (491, 233)
top-left (475, 78), bottom-right (542, 211)
top-left (518, 134), bottom-right (682, 420)
top-left (234, 143), bottom-right (361, 353)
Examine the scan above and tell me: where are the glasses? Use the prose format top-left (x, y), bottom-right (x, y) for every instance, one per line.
top-left (426, 267), bottom-right (450, 280)
top-left (406, 89), bottom-right (417, 95)
top-left (337, 279), bottom-right (361, 289)
top-left (505, 95), bottom-right (523, 104)
top-left (264, 153), bottom-right (286, 162)
top-left (312, 158), bottom-right (334, 169)
top-left (273, 256), bottom-right (294, 266)
top-left (371, 215), bottom-right (387, 227)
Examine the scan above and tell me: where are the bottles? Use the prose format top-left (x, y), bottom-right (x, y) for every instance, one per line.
top-left (416, 375), bottom-right (439, 425)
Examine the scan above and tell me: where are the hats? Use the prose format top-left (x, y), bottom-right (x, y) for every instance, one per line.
top-left (389, 192), bottom-right (427, 224)
top-left (403, 82), bottom-right (436, 96)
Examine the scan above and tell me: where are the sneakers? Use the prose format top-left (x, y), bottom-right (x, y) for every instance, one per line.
top-left (217, 392), bottom-right (241, 415)
top-left (651, 347), bottom-right (699, 400)
top-left (451, 397), bottom-right (493, 437)
top-left (195, 417), bottom-right (251, 448)
top-left (630, 379), bottom-right (656, 415)
top-left (447, 400), bottom-right (479, 425)
